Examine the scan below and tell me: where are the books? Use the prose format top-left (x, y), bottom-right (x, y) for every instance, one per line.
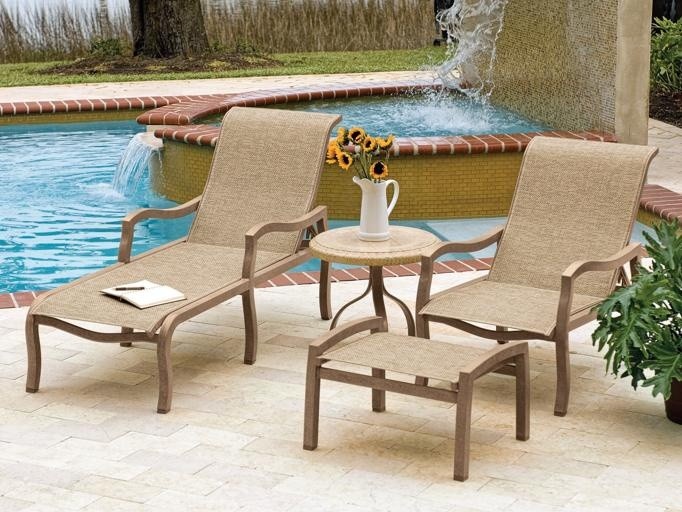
top-left (98, 279), bottom-right (187, 310)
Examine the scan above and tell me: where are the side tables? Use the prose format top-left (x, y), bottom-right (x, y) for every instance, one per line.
top-left (307, 225), bottom-right (443, 337)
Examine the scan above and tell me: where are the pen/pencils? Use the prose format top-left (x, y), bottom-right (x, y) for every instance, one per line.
top-left (116, 287), bottom-right (144, 290)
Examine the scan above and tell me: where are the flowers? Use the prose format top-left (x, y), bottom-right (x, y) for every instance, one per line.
top-left (324, 126), bottom-right (396, 184)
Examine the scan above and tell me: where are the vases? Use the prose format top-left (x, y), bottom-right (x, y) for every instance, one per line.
top-left (352, 175), bottom-right (400, 242)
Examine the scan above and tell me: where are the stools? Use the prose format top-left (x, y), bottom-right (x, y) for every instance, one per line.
top-left (303, 315), bottom-right (531, 482)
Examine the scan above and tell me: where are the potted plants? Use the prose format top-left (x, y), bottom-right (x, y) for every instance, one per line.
top-left (588, 215), bottom-right (682, 425)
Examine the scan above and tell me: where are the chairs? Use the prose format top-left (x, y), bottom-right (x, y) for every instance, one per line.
top-left (415, 135), bottom-right (659, 418)
top-left (24, 105), bottom-right (342, 414)
